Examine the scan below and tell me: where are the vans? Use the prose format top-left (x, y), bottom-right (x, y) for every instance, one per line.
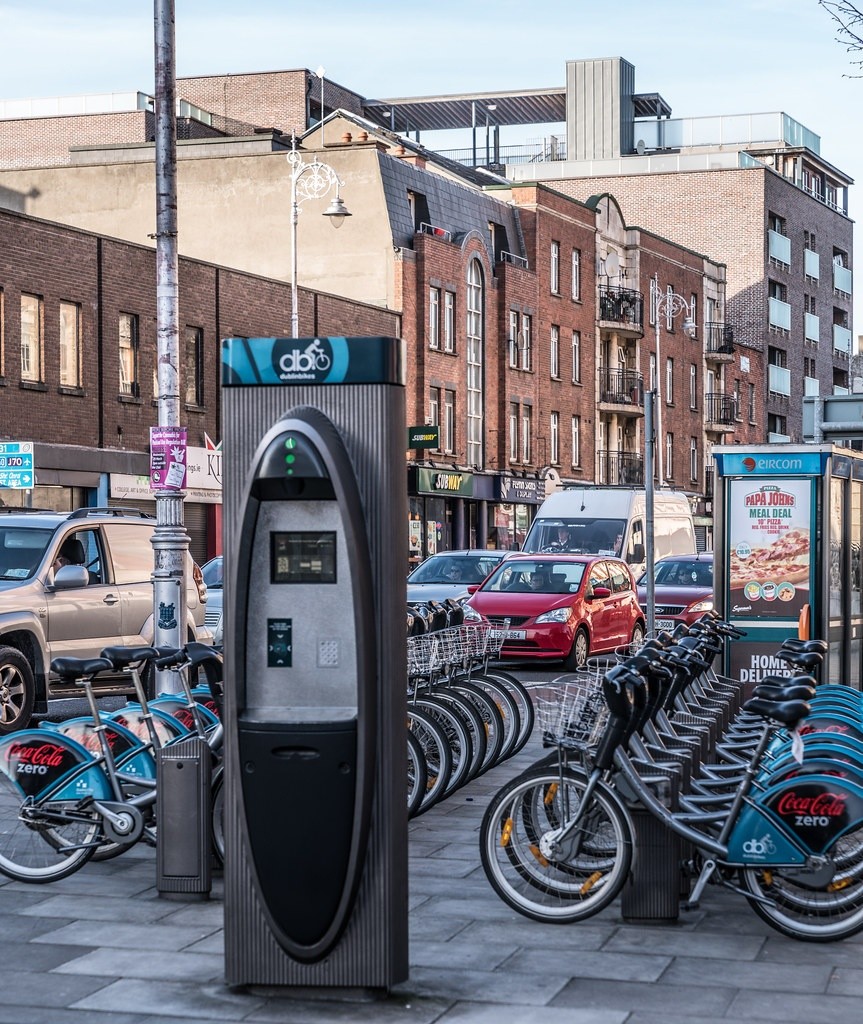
top-left (521, 484), bottom-right (699, 582)
top-left (630, 552), bottom-right (715, 649)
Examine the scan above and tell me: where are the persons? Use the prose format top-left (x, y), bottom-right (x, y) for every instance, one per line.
top-left (29, 548), bottom-right (100, 586)
top-left (550, 527), bottom-right (581, 554)
top-left (610, 531), bottom-right (625, 556)
top-left (676, 569), bottom-right (696, 585)
top-left (444, 565), bottom-right (462, 583)
top-left (527, 572), bottom-right (546, 593)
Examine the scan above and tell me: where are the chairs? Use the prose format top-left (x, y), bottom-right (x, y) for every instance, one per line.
top-left (61, 539), bottom-right (103, 587)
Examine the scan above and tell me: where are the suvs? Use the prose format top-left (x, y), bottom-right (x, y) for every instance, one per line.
top-left (0, 505), bottom-right (209, 736)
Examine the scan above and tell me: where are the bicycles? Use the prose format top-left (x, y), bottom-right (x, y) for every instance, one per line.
top-left (0, 599), bottom-right (536, 888)
top-left (476, 607), bottom-right (863, 944)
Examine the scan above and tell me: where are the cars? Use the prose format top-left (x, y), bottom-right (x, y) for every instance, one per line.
top-left (199, 552), bottom-right (225, 652)
top-left (406, 548), bottom-right (533, 611)
top-left (464, 552), bottom-right (650, 671)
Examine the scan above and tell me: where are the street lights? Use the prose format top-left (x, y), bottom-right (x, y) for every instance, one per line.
top-left (649, 273), bottom-right (699, 492)
top-left (286, 128), bottom-right (353, 345)
